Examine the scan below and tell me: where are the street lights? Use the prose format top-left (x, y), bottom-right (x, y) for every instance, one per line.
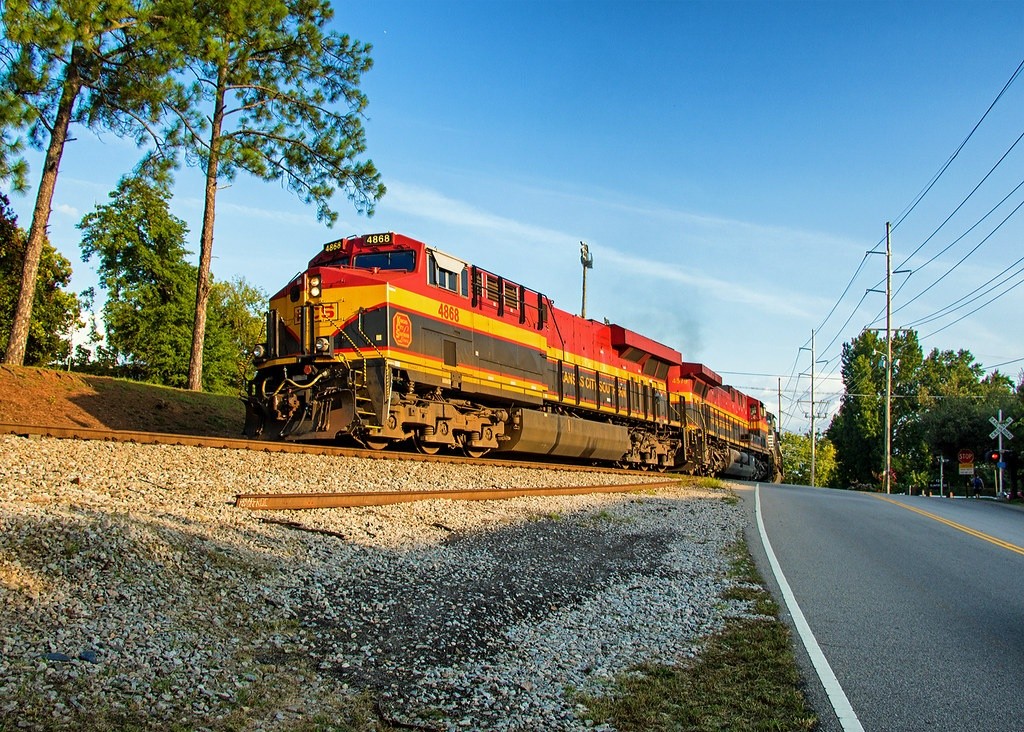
top-left (579, 241), bottom-right (593, 316)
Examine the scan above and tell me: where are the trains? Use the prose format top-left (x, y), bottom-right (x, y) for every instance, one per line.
top-left (238, 230), bottom-right (785, 485)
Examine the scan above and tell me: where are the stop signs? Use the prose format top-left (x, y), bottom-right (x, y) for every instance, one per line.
top-left (957, 448), bottom-right (974, 465)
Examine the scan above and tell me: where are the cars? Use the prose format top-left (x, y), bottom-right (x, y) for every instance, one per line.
top-left (1000, 491), bottom-right (1024, 502)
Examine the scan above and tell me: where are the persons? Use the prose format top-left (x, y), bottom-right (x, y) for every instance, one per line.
top-left (971, 473), bottom-right (984, 499)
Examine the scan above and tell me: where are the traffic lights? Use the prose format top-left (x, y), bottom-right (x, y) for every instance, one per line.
top-left (991, 451), bottom-right (1000, 460)
